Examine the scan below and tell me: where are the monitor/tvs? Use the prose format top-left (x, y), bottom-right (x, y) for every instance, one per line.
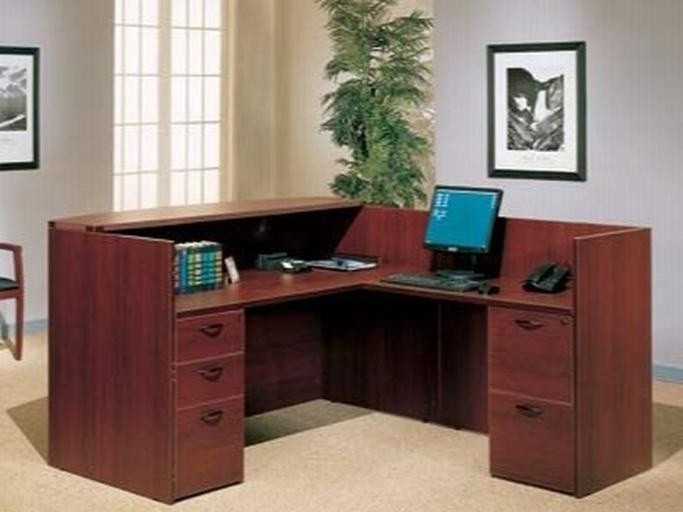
top-left (421, 184), bottom-right (503, 280)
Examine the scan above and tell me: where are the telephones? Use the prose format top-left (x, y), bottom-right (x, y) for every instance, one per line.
top-left (522, 263), bottom-right (570, 293)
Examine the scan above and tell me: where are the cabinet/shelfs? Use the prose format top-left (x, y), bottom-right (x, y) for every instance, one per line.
top-left (482, 306), bottom-right (576, 500)
top-left (167, 307), bottom-right (244, 505)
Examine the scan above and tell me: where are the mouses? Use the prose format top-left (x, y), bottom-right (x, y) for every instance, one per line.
top-left (476, 284), bottom-right (500, 296)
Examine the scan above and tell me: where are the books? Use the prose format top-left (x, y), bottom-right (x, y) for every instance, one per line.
top-left (172, 240), bottom-right (225, 296)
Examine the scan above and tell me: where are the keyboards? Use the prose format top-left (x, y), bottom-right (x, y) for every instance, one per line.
top-left (380, 271), bottom-right (480, 293)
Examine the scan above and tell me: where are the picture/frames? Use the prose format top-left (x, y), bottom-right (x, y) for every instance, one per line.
top-left (0, 45), bottom-right (40, 173)
top-left (484, 40), bottom-right (589, 184)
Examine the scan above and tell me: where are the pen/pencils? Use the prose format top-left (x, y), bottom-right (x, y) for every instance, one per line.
top-left (332, 257), bottom-right (348, 268)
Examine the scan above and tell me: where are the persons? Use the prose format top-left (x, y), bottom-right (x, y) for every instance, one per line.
top-left (508, 69), bottom-right (565, 151)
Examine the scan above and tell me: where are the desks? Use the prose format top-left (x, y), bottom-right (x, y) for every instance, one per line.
top-left (44, 200), bottom-right (654, 506)
